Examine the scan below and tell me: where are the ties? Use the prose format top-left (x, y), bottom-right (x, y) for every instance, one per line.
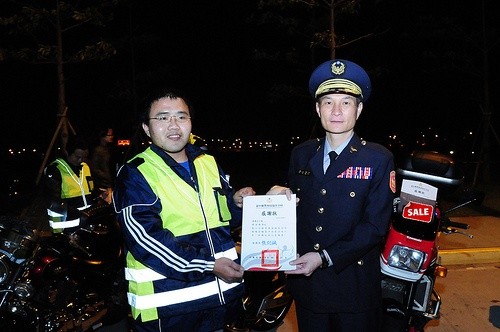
top-left (329, 151), bottom-right (338, 165)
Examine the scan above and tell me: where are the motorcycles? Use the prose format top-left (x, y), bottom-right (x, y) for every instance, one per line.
top-left (0, 193), bottom-right (294, 332)
top-left (362, 146), bottom-right (478, 332)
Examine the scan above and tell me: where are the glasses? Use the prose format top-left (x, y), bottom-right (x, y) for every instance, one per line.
top-left (149, 115), bottom-right (191, 123)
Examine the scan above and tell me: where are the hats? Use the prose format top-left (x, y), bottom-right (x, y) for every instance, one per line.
top-left (310, 59), bottom-right (372, 103)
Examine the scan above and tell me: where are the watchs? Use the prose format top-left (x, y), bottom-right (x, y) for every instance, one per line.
top-left (319, 251), bottom-right (328, 269)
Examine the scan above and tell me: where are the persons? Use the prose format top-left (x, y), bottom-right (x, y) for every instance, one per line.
top-left (108, 88), bottom-right (257, 332)
top-left (265, 59), bottom-right (396, 332)
top-left (39, 124), bottom-right (121, 234)
